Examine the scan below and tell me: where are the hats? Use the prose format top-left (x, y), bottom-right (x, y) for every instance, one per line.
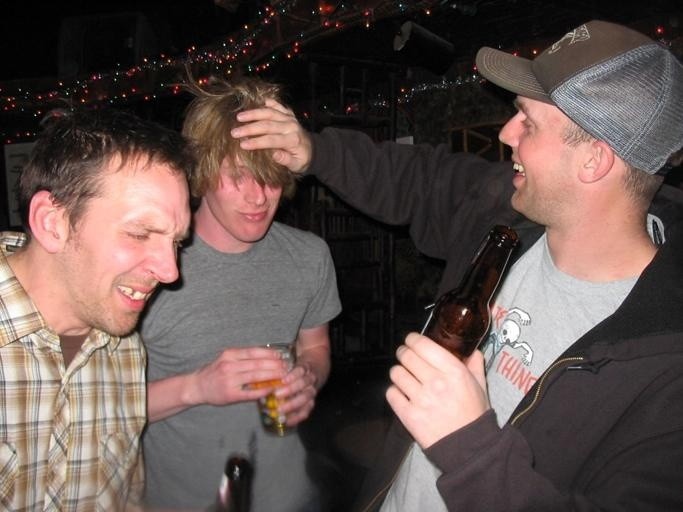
top-left (475, 20), bottom-right (682, 176)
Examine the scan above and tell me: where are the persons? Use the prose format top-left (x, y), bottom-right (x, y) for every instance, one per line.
top-left (0, 108), bottom-right (191, 512)
top-left (139, 82), bottom-right (343, 511)
top-left (231, 21), bottom-right (683, 512)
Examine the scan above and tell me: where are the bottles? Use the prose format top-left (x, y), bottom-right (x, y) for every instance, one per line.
top-left (215, 455), bottom-right (255, 512)
top-left (419, 224), bottom-right (517, 364)
